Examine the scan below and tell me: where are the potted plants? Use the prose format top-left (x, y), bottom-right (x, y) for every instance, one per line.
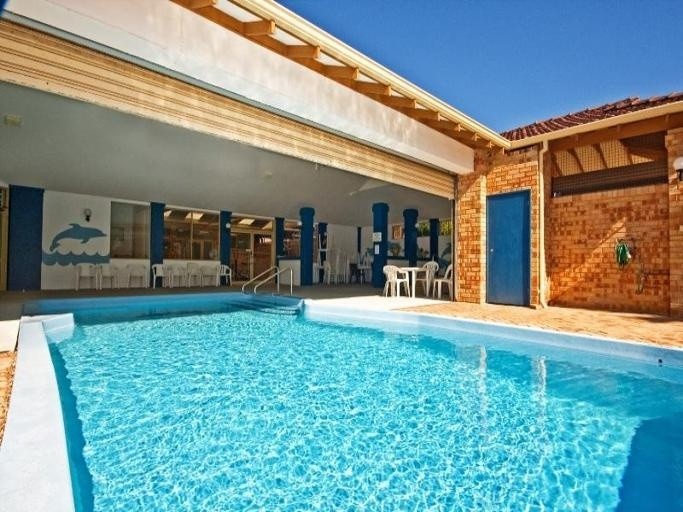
top-left (390, 243), bottom-right (402, 256)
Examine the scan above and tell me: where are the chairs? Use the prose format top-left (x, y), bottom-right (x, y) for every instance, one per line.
top-left (74, 262), bottom-right (232, 292)
top-left (382, 260), bottom-right (452, 299)
top-left (322, 259), bottom-right (366, 288)
